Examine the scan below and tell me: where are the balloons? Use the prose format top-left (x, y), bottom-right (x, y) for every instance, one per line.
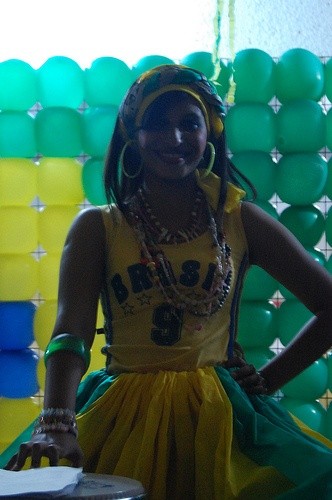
top-left (0, 397), bottom-right (43, 455)
top-left (0, 55), bottom-right (175, 208)
top-left (234, 201), bottom-right (332, 444)
top-left (180, 48), bottom-right (332, 206)
top-left (0, 204), bottom-right (108, 399)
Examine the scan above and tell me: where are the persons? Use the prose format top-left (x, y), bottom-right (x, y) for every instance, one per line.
top-left (0, 64), bottom-right (332, 500)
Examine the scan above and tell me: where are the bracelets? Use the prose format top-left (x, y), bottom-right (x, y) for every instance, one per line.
top-left (32, 408), bottom-right (79, 439)
top-left (44, 333), bottom-right (91, 374)
top-left (256, 369), bottom-right (274, 395)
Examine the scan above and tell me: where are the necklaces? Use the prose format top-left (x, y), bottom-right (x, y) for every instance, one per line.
top-left (121, 182), bottom-right (233, 332)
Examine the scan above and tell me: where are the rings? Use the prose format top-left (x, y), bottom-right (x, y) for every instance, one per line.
top-left (255, 375), bottom-right (264, 385)
